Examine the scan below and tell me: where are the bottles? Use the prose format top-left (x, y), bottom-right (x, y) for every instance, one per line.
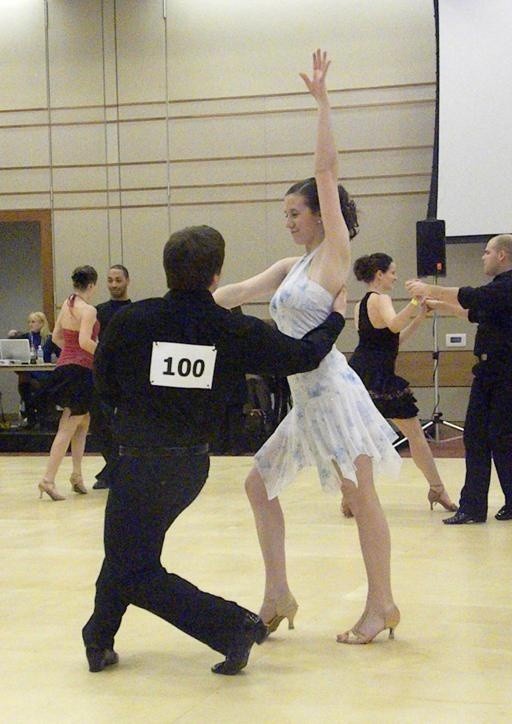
top-left (30, 344), bottom-right (36, 364)
top-left (37, 346), bottom-right (44, 364)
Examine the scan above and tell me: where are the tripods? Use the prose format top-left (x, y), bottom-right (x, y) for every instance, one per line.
top-left (392, 276), bottom-right (464, 448)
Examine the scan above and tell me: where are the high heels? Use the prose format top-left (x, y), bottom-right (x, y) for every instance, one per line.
top-left (70, 474), bottom-right (89, 494)
top-left (429, 483), bottom-right (458, 512)
top-left (256, 593), bottom-right (298, 635)
top-left (337, 605), bottom-right (400, 643)
top-left (38, 479), bottom-right (66, 501)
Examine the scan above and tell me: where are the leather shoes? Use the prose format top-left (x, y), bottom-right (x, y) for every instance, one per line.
top-left (85, 646), bottom-right (119, 672)
top-left (495, 506), bottom-right (511, 520)
top-left (442, 511), bottom-right (486, 524)
top-left (211, 613), bottom-right (267, 674)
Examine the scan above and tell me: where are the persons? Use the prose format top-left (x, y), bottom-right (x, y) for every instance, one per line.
top-left (82, 225), bottom-right (347, 675)
top-left (404, 233), bottom-right (512, 524)
top-left (8, 311), bottom-right (62, 429)
top-left (341, 253), bottom-right (458, 518)
top-left (211, 49), bottom-right (401, 645)
top-left (93, 264), bottom-right (134, 489)
top-left (38, 265), bottom-right (100, 501)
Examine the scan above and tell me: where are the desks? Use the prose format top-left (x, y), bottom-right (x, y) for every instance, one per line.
top-left (1, 363), bottom-right (63, 450)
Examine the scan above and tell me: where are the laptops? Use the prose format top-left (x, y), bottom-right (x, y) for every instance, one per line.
top-left (0, 339), bottom-right (30, 364)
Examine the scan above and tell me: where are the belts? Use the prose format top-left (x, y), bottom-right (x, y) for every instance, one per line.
top-left (117, 444), bottom-right (209, 460)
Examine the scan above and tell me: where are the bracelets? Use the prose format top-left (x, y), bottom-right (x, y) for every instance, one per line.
top-left (411, 299), bottom-right (418, 306)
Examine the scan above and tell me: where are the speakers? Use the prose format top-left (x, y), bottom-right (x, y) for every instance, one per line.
top-left (416, 220), bottom-right (446, 279)
